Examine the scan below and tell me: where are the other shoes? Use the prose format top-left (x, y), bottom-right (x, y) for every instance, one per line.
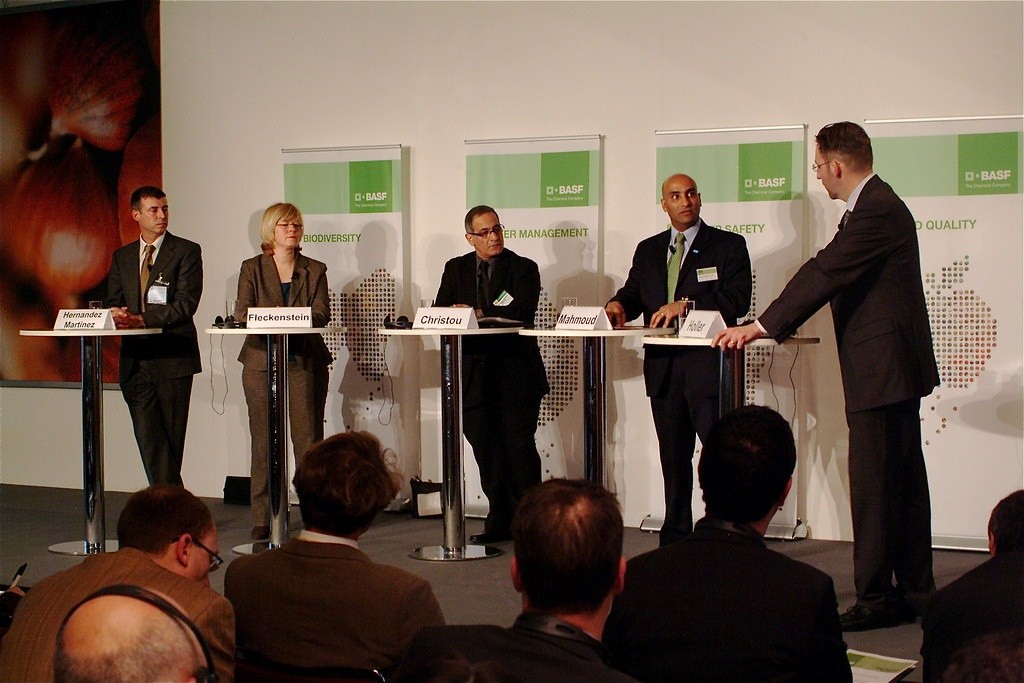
top-left (252, 526), bottom-right (269, 539)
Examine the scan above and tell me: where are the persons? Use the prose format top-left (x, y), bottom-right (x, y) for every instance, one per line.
top-left (234, 203), bottom-right (335, 543)
top-left (710, 122), bottom-right (942, 630)
top-left (224, 430), bottom-right (446, 672)
top-left (0, 482), bottom-right (236, 683)
top-left (603, 175), bottom-right (753, 551)
top-left (51, 582), bottom-right (220, 683)
top-left (919, 490), bottom-right (1024, 683)
top-left (0, 586), bottom-right (26, 638)
top-left (433, 205), bottom-right (551, 544)
top-left (388, 476), bottom-right (643, 683)
top-left (600, 405), bottom-right (854, 683)
top-left (101, 186), bottom-right (205, 487)
top-left (935, 615), bottom-right (1024, 682)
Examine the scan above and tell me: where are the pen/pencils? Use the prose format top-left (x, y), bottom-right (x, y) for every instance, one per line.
top-left (9, 563), bottom-right (27, 589)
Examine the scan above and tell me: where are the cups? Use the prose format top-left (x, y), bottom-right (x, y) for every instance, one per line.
top-left (226, 299), bottom-right (239, 327)
top-left (678, 301), bottom-right (695, 334)
top-left (88, 301), bottom-right (102, 309)
top-left (562, 298), bottom-right (577, 308)
top-left (421, 299), bottom-right (434, 307)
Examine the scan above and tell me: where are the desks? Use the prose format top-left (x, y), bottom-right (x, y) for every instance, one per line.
top-left (18, 328), bottom-right (163, 555)
top-left (644, 334), bottom-right (822, 421)
top-left (204, 327), bottom-right (347, 556)
top-left (378, 328), bottom-right (526, 560)
top-left (519, 326), bottom-right (674, 485)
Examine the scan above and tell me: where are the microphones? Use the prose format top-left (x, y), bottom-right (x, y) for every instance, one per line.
top-left (669, 245), bottom-right (675, 253)
top-left (147, 264), bottom-right (152, 272)
top-left (838, 224), bottom-right (844, 231)
top-left (292, 272), bottom-right (299, 278)
top-left (477, 274), bottom-right (481, 280)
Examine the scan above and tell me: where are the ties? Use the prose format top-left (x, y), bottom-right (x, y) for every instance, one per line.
top-left (140, 245), bottom-right (155, 313)
top-left (843, 210), bottom-right (850, 230)
top-left (478, 261), bottom-right (490, 316)
top-left (668, 233), bottom-right (685, 304)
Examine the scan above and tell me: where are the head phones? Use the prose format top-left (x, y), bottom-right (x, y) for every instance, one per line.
top-left (384, 315), bottom-right (413, 329)
top-left (212, 315), bottom-right (247, 329)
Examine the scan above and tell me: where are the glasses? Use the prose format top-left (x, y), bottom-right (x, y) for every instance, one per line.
top-left (170, 536), bottom-right (224, 571)
top-left (277, 224), bottom-right (303, 230)
top-left (812, 160), bottom-right (841, 172)
top-left (468, 224), bottom-right (505, 238)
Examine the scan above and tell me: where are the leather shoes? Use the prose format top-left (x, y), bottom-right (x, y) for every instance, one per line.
top-left (470, 531), bottom-right (511, 543)
top-left (839, 604), bottom-right (899, 631)
top-left (898, 599), bottom-right (923, 624)
